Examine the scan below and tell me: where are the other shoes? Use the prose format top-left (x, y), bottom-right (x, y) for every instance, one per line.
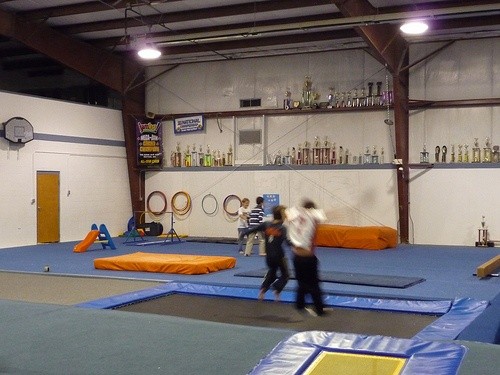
top-left (240, 251), bottom-right (244, 254)
top-left (244, 254), bottom-right (250, 256)
top-left (260, 253), bottom-right (266, 255)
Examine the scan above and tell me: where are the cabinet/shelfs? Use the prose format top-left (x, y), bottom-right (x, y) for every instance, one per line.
top-left (142, 98), bottom-right (500, 171)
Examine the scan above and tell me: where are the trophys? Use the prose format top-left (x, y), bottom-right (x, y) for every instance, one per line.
top-left (283, 74), bottom-right (393, 111)
top-left (420, 138), bottom-right (500, 164)
top-left (477, 216), bottom-right (489, 247)
top-left (266, 136), bottom-right (385, 166)
top-left (171, 144), bottom-right (233, 167)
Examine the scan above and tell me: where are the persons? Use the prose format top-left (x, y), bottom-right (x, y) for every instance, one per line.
top-left (244, 196), bottom-right (267, 256)
top-left (282, 201), bottom-right (333, 317)
top-left (237, 197), bottom-right (254, 255)
top-left (239, 205), bottom-right (290, 302)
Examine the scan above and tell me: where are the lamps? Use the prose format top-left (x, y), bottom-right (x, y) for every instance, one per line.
top-left (137, 24), bottom-right (162, 60)
